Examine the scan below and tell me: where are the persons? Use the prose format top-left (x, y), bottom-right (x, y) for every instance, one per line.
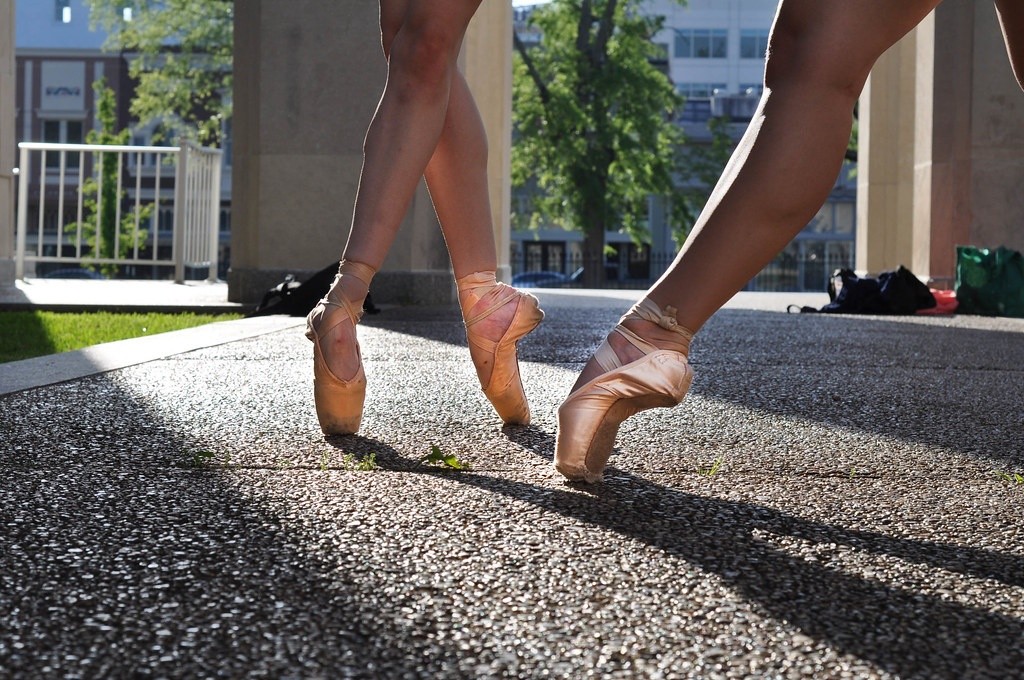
top-left (305, 0), bottom-right (545, 435)
top-left (553, 0), bottom-right (1024, 484)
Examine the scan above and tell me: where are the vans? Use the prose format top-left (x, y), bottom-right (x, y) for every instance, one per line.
top-left (569, 262), bottom-right (646, 285)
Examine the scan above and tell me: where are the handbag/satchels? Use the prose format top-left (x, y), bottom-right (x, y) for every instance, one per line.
top-left (954, 244), bottom-right (1024, 318)
top-left (248, 260), bottom-right (381, 317)
top-left (787, 264), bottom-right (937, 316)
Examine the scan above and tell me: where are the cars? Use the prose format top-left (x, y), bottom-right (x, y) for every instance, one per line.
top-left (513, 271), bottom-right (571, 289)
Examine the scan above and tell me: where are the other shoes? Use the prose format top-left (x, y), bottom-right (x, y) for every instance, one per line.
top-left (554, 298), bottom-right (693, 484)
top-left (457, 272), bottom-right (545, 427)
top-left (305, 265), bottom-right (367, 435)
